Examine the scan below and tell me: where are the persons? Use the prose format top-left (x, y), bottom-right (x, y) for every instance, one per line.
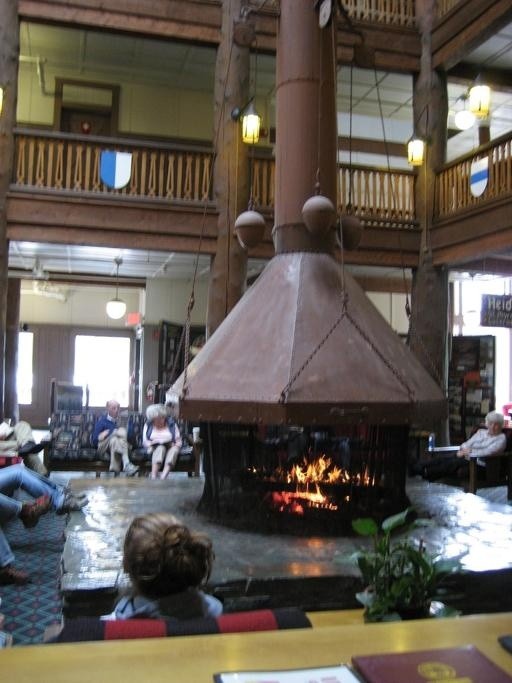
top-left (92, 399), bottom-right (140, 477)
top-left (0, 489), bottom-right (53, 585)
top-left (142, 403), bottom-right (184, 478)
top-left (0, 421), bottom-right (54, 477)
top-left (424, 410), bottom-right (506, 482)
top-left (98, 512), bottom-right (224, 621)
top-left (0, 463), bottom-right (90, 515)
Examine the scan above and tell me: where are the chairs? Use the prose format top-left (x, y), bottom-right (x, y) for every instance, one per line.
top-left (42, 410), bottom-right (203, 478)
top-left (424, 426), bottom-right (512, 494)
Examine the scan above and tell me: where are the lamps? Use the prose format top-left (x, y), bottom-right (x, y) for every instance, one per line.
top-left (405, 104), bottom-right (432, 166)
top-left (453, 67), bottom-right (489, 128)
top-left (230, 96), bottom-right (263, 144)
top-left (106, 256), bottom-right (128, 319)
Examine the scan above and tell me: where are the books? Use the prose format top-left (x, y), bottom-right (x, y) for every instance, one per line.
top-left (352, 644), bottom-right (510, 682)
top-left (214, 664), bottom-right (364, 682)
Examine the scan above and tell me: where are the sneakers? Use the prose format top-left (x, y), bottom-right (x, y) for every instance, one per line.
top-left (0, 567), bottom-right (28, 585)
top-left (19, 493), bottom-right (52, 528)
top-left (124, 463), bottom-right (140, 476)
top-left (57, 491), bottom-right (89, 514)
top-left (19, 442), bottom-right (44, 455)
